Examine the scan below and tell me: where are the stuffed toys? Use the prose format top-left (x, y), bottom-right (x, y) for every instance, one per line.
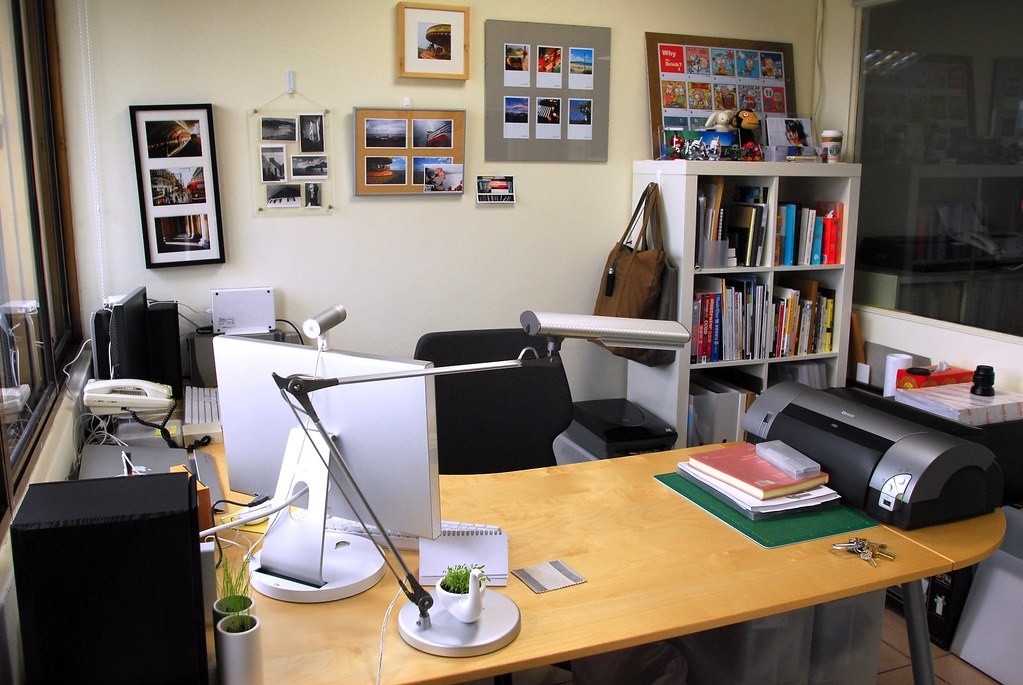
top-left (705, 108), bottom-right (760, 149)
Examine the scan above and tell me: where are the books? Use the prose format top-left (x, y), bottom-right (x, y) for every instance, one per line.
top-left (696, 177), bottom-right (769, 267)
top-left (677, 443), bottom-right (840, 520)
top-left (768, 277), bottom-right (836, 357)
top-left (774, 201), bottom-right (845, 266)
top-left (691, 275), bottom-right (769, 365)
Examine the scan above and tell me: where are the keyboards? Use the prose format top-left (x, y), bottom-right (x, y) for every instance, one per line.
top-left (183, 385), bottom-right (223, 427)
top-left (322, 512), bottom-right (501, 551)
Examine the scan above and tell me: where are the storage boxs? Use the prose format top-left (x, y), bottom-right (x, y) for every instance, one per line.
top-left (693, 376), bottom-right (739, 443)
top-left (688, 393), bottom-right (714, 448)
top-left (703, 236), bottom-right (729, 268)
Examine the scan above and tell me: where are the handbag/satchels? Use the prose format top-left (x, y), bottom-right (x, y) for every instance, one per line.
top-left (588, 181), bottom-right (680, 368)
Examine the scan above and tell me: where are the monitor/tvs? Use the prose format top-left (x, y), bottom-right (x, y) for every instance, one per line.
top-left (212, 335), bottom-right (443, 603)
top-left (108, 285), bottom-right (148, 382)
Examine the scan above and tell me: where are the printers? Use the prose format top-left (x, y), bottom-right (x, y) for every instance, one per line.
top-left (739, 380), bottom-right (1004, 530)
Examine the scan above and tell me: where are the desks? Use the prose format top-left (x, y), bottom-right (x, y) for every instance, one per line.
top-left (208, 439), bottom-right (1009, 685)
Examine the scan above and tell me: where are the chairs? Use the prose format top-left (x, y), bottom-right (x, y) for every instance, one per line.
top-left (413, 327), bottom-right (575, 479)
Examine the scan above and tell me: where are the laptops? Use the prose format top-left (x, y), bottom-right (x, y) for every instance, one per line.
top-left (78, 442), bottom-right (193, 479)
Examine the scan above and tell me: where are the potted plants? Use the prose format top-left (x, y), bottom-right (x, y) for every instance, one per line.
top-left (435, 558), bottom-right (493, 621)
top-left (209, 547), bottom-right (266, 685)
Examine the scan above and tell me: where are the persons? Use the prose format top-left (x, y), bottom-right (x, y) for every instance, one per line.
top-left (785, 119), bottom-right (808, 146)
top-left (431, 167), bottom-right (463, 191)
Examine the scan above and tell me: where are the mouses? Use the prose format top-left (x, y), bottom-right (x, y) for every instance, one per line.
top-left (238, 500), bottom-right (272, 525)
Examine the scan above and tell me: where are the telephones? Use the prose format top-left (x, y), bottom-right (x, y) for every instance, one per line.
top-left (83, 378), bottom-right (175, 416)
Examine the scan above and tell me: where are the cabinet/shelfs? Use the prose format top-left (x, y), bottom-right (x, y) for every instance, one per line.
top-left (626, 160), bottom-right (865, 441)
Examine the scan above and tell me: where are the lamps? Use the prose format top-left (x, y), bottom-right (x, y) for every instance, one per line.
top-left (269, 308), bottom-right (692, 656)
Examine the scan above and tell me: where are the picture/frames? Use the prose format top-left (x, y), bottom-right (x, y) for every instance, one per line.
top-left (351, 104), bottom-right (467, 199)
top-left (249, 106), bottom-right (335, 216)
top-left (126, 102), bottom-right (227, 270)
top-left (393, 1), bottom-right (472, 82)
top-left (641, 28), bottom-right (799, 159)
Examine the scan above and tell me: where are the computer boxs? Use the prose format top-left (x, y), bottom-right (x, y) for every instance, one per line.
top-left (188, 328), bottom-right (286, 389)
top-left (93, 300), bottom-right (184, 399)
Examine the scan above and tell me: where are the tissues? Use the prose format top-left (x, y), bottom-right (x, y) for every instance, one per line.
top-left (896, 361), bottom-right (974, 389)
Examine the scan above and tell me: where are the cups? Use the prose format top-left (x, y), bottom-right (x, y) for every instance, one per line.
top-left (821, 130), bottom-right (844, 163)
